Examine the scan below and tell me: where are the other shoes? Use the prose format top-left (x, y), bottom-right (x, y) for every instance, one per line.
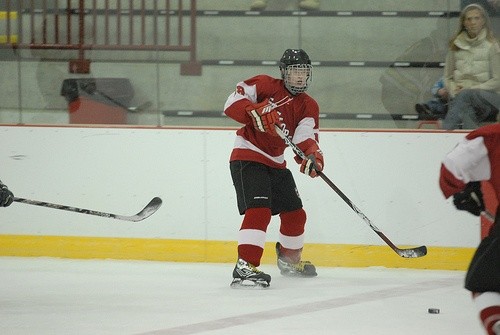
top-left (416, 103), bottom-right (437, 120)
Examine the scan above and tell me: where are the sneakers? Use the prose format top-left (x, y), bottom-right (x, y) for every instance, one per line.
top-left (277, 257), bottom-right (319, 279)
top-left (229, 259), bottom-right (272, 291)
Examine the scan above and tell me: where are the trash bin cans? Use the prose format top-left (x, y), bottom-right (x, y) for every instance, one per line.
top-left (59, 77), bottom-right (135, 124)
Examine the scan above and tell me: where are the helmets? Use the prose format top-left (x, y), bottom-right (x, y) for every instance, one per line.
top-left (280, 48), bottom-right (315, 95)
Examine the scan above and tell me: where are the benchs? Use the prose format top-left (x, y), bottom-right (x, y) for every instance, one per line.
top-left (0, 0), bottom-right (500, 128)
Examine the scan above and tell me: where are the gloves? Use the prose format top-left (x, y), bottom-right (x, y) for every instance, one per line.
top-left (294, 151), bottom-right (325, 178)
top-left (247, 99), bottom-right (284, 138)
top-left (454, 186), bottom-right (486, 217)
top-left (0, 179), bottom-right (15, 208)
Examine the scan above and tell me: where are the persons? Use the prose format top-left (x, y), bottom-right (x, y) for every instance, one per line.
top-left (30, 20), bottom-right (97, 124)
top-left (379, 12), bottom-right (461, 129)
top-left (431, 3), bottom-right (500, 335)
top-left (0, 180), bottom-right (14, 208)
top-left (224, 49), bottom-right (324, 289)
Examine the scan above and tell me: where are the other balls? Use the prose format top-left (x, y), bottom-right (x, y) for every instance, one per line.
top-left (428, 308), bottom-right (440, 314)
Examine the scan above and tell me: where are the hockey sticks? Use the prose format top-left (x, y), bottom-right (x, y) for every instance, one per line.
top-left (274, 124), bottom-right (428, 259)
top-left (95, 86), bottom-right (156, 114)
top-left (12, 197), bottom-right (163, 224)
